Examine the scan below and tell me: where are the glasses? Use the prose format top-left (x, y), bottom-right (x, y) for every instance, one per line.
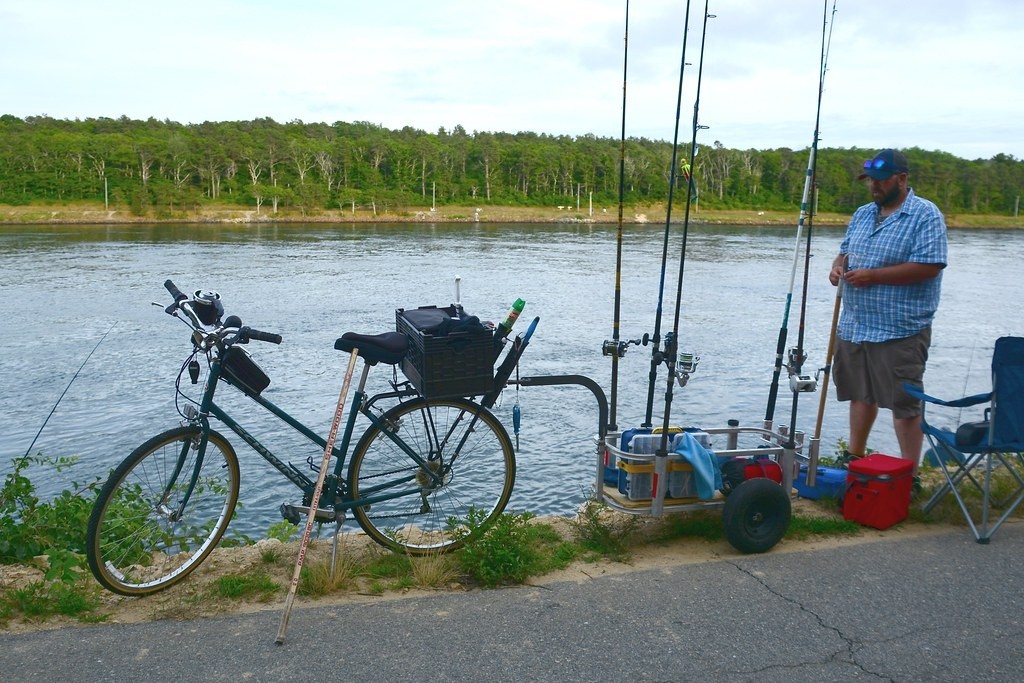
top-left (863, 159), bottom-right (889, 171)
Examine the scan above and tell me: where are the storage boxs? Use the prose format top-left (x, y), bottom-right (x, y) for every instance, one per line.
top-left (792, 464), bottom-right (851, 501)
top-left (395, 305), bottom-right (495, 399)
top-left (843, 454), bottom-right (917, 529)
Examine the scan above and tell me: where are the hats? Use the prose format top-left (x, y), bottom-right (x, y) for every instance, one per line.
top-left (858, 148), bottom-right (910, 180)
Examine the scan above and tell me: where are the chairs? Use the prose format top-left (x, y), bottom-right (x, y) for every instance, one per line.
top-left (902, 338), bottom-right (1024, 545)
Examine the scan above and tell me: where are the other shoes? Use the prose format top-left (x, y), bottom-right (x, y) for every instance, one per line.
top-left (833, 449), bottom-right (863, 471)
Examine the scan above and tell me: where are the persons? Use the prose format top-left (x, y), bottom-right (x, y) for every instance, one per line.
top-left (829, 149), bottom-right (948, 501)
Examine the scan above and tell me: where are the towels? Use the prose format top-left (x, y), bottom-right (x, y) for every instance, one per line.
top-left (673, 431), bottom-right (725, 500)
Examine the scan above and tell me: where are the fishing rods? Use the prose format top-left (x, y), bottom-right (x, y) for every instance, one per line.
top-left (605, 0), bottom-right (850, 499)
top-left (10, 319), bottom-right (120, 484)
top-left (955, 338), bottom-right (978, 429)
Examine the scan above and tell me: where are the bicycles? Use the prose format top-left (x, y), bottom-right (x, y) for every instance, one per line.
top-left (85, 281), bottom-right (516, 597)
